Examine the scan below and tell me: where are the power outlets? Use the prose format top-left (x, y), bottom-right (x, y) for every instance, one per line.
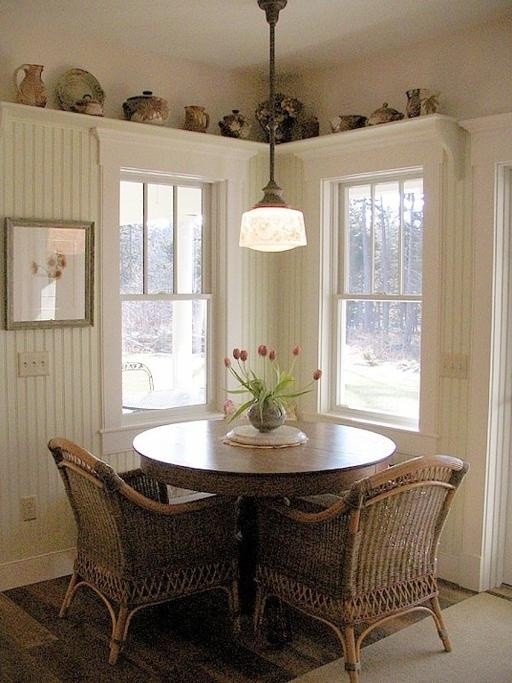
top-left (19, 496), bottom-right (39, 521)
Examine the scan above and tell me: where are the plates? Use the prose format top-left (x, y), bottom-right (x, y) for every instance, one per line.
top-left (226, 424), bottom-right (309, 446)
top-left (55, 68), bottom-right (106, 114)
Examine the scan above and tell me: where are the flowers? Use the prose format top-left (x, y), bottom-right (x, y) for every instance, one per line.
top-left (200, 342), bottom-right (324, 423)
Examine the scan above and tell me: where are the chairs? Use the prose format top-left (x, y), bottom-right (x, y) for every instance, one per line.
top-left (236, 453), bottom-right (470, 682)
top-left (48, 436), bottom-right (248, 666)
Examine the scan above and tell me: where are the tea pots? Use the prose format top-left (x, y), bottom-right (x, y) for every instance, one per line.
top-left (183, 105), bottom-right (210, 133)
top-left (84, 100), bottom-right (104, 116)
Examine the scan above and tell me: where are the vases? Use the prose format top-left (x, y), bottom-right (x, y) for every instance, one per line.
top-left (248, 397), bottom-right (288, 435)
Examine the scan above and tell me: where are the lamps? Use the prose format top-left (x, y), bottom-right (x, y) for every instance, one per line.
top-left (238, 0), bottom-right (309, 256)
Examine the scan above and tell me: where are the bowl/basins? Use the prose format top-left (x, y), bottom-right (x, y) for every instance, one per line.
top-left (327, 115), bottom-right (367, 133)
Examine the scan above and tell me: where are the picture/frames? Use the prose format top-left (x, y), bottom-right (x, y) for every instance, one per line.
top-left (4, 218), bottom-right (95, 331)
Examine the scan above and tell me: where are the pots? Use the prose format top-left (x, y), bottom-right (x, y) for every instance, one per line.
top-left (219, 109), bottom-right (251, 140)
top-left (120, 90), bottom-right (170, 125)
top-left (367, 102), bottom-right (404, 125)
